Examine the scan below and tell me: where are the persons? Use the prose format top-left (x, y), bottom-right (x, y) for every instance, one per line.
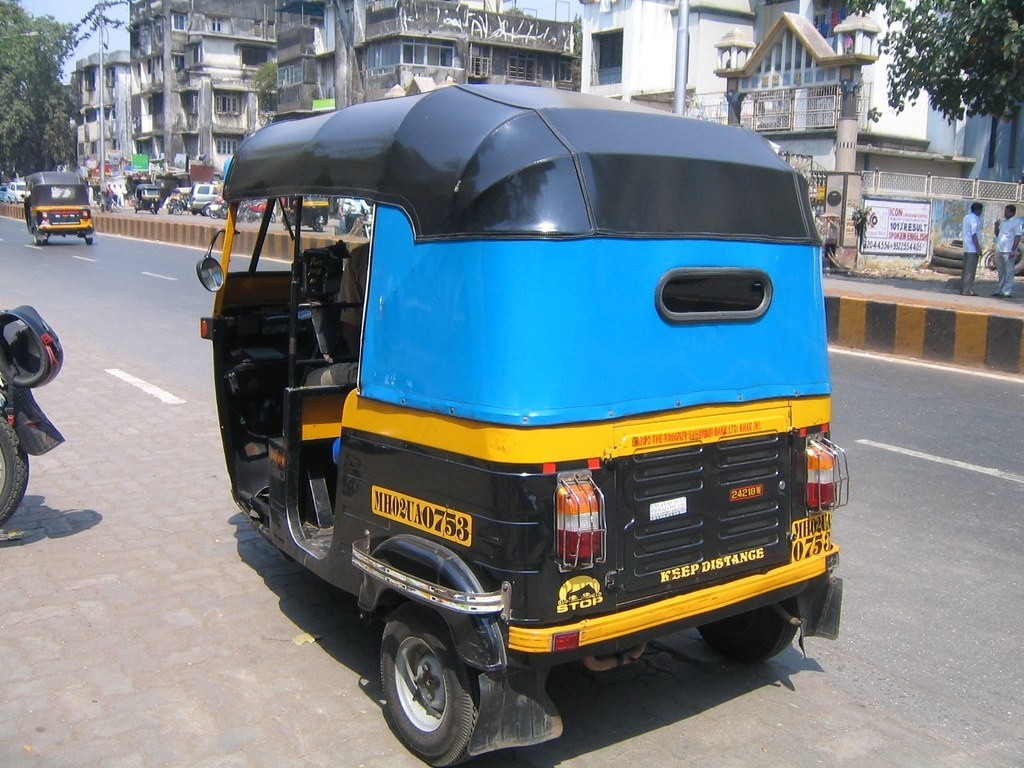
top-left (304, 242), bottom-right (369, 384)
top-left (961, 202), bottom-right (983, 295)
top-left (992, 204), bottom-right (1022, 298)
top-left (338, 197), bottom-right (369, 231)
top-left (107, 184), bottom-right (123, 211)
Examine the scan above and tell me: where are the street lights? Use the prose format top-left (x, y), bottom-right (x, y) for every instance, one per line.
top-left (98, 10), bottom-right (110, 212)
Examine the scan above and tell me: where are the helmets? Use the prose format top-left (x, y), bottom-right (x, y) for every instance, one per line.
top-left (0, 305), bottom-right (63, 388)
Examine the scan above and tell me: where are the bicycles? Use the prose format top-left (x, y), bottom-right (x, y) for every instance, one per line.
top-left (168, 202), bottom-right (184, 215)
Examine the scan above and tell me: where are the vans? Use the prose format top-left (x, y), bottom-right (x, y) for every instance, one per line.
top-left (190, 184), bottom-right (220, 215)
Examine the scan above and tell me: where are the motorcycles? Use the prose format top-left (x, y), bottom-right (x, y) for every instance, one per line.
top-left (0, 306), bottom-right (65, 525)
top-left (283, 194), bottom-right (330, 231)
top-left (134, 184), bottom-right (161, 216)
top-left (197, 82), bottom-right (849, 767)
top-left (218, 200), bottom-right (276, 223)
top-left (23, 172), bottom-right (95, 246)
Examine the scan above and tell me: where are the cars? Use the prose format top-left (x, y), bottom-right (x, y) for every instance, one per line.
top-left (0, 181), bottom-right (30, 203)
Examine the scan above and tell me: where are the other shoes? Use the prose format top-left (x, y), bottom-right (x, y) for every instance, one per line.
top-left (961, 289), bottom-right (978, 296)
top-left (992, 293), bottom-right (1003, 296)
top-left (1000, 294), bottom-right (1010, 298)
